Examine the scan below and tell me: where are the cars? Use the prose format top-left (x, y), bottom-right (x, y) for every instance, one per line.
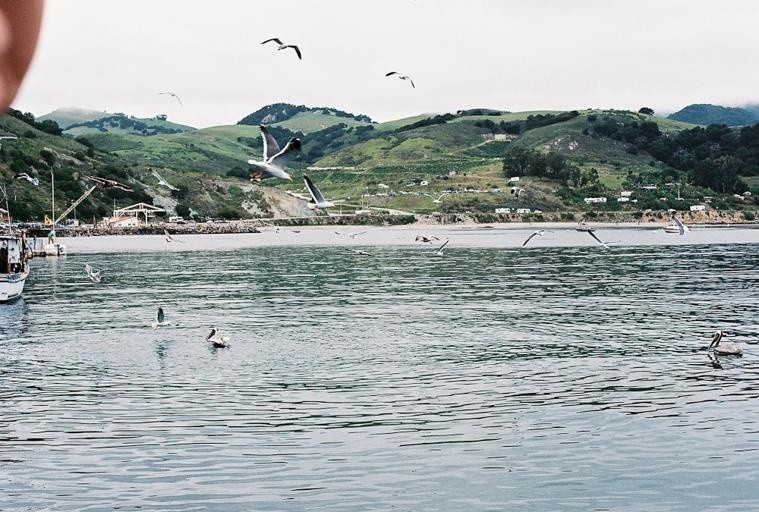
top-left (0, 219), bottom-right (146, 236)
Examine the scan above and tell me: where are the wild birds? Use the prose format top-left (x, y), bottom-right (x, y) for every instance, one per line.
top-left (248, 124), bottom-right (302, 182)
top-left (671, 215), bottom-right (691, 236)
top-left (707, 330), bottom-right (743, 354)
top-left (85, 264), bottom-right (106, 284)
top-left (258, 218), bottom-right (300, 234)
top-left (17, 172), bottom-right (39, 186)
top-left (148, 167), bottom-right (180, 192)
top-left (415, 236), bottom-right (440, 245)
top-left (335, 230), bottom-right (368, 240)
top-left (303, 172), bottom-right (337, 211)
top-left (510, 186), bottom-right (526, 200)
top-left (587, 229), bottom-right (621, 250)
top-left (386, 71), bottom-right (415, 89)
top-left (204, 327), bottom-right (232, 348)
top-left (424, 193), bottom-right (450, 203)
top-left (260, 37), bottom-right (302, 60)
top-left (157, 308), bottom-right (164, 323)
top-left (522, 229), bottom-right (555, 246)
top-left (421, 240), bottom-right (450, 256)
top-left (164, 229), bottom-right (185, 245)
top-left (160, 91), bottom-right (183, 107)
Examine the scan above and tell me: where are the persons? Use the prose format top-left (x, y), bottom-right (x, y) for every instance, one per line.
top-left (46, 230), bottom-right (54, 243)
top-left (0, 0), bottom-right (47, 114)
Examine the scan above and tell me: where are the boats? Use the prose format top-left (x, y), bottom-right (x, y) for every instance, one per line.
top-left (664, 217), bottom-right (692, 234)
top-left (577, 218), bottom-right (597, 232)
top-left (0, 236), bottom-right (29, 306)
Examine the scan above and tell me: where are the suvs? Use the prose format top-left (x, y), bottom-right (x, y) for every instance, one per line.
top-left (169, 215), bottom-right (187, 223)
